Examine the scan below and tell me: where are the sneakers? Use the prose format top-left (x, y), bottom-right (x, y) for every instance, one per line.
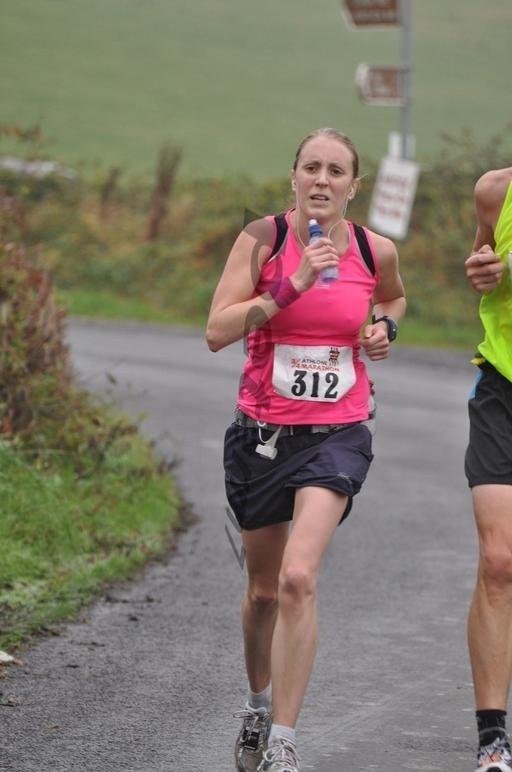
top-left (260, 732), bottom-right (302, 772)
top-left (474, 725), bottom-right (512, 771)
top-left (232, 700), bottom-right (273, 772)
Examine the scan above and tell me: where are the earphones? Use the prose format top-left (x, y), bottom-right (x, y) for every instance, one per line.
top-left (291, 180), bottom-right (297, 187)
top-left (349, 187), bottom-right (354, 196)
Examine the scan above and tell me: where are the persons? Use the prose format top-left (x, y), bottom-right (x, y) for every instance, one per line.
top-left (200, 122), bottom-right (406, 772)
top-left (456, 164), bottom-right (512, 772)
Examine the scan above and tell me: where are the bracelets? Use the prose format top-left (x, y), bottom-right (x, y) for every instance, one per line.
top-left (267, 275), bottom-right (301, 313)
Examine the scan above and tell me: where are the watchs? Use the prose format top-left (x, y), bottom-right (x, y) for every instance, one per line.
top-left (371, 312), bottom-right (398, 347)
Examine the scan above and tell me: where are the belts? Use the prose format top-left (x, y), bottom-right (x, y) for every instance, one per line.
top-left (235, 406), bottom-right (356, 440)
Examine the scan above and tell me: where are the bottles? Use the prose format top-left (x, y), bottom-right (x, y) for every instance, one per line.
top-left (308, 217), bottom-right (339, 283)
